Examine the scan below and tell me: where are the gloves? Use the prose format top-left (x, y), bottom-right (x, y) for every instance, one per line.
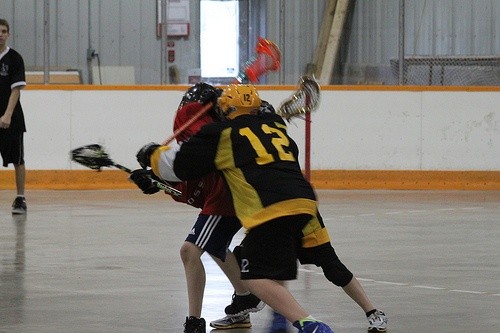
top-left (130, 169), bottom-right (165, 194)
top-left (137, 143), bottom-right (161, 169)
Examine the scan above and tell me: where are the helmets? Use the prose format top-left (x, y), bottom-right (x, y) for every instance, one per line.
top-left (179, 82), bottom-right (219, 119)
top-left (217, 84), bottom-right (260, 120)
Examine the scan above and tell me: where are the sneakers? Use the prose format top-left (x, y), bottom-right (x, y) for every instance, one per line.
top-left (225, 292), bottom-right (264, 317)
top-left (12, 196), bottom-right (27, 214)
top-left (292, 319), bottom-right (334, 333)
top-left (184, 315), bottom-right (206, 333)
top-left (210, 313), bottom-right (252, 329)
top-left (368, 309), bottom-right (387, 332)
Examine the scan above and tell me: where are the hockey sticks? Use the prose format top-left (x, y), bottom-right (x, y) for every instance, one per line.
top-left (161, 36), bottom-right (281, 148)
top-left (267, 90), bottom-right (312, 333)
top-left (71, 143), bottom-right (182, 198)
top-left (276, 73), bottom-right (321, 118)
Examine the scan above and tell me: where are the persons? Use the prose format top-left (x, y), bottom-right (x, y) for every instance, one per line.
top-left (129, 83), bottom-right (266, 333)
top-left (136, 81), bottom-right (336, 333)
top-left (209, 208), bottom-right (388, 333)
top-left (0, 19), bottom-right (27, 214)
